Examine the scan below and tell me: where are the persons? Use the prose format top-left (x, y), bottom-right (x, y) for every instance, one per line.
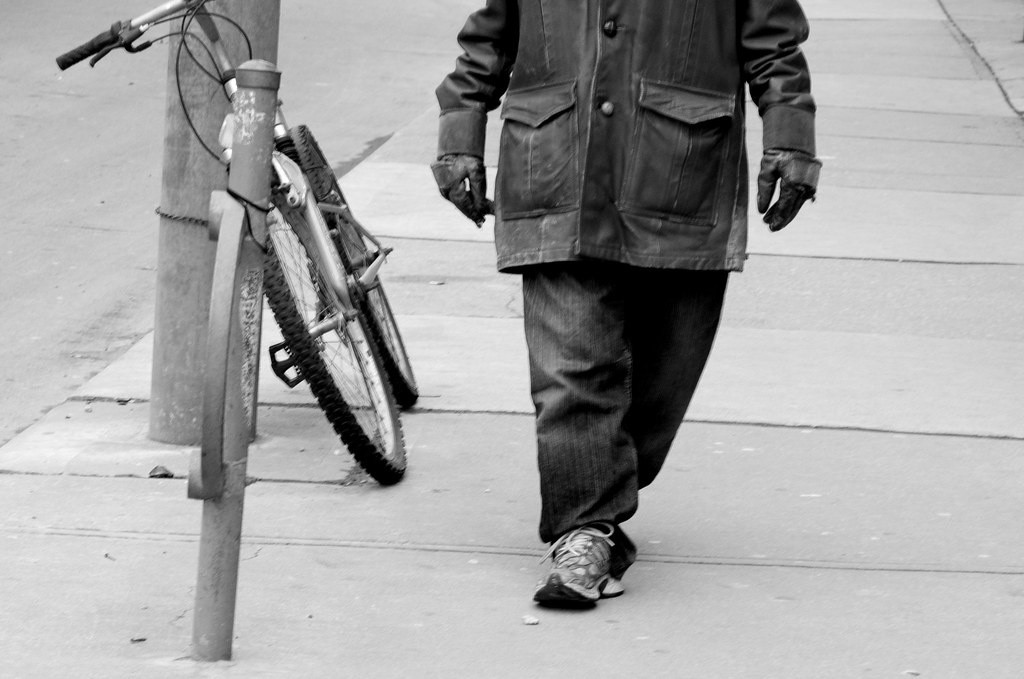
top-left (430, 0), bottom-right (823, 603)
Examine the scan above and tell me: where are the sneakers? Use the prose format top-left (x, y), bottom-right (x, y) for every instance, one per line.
top-left (534, 522), bottom-right (626, 607)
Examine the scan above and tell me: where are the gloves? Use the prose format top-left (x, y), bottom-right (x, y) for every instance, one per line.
top-left (431, 152), bottom-right (497, 228)
top-left (757, 148), bottom-right (823, 231)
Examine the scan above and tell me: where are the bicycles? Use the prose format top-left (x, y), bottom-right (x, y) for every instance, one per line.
top-left (56, 1), bottom-right (418, 485)
